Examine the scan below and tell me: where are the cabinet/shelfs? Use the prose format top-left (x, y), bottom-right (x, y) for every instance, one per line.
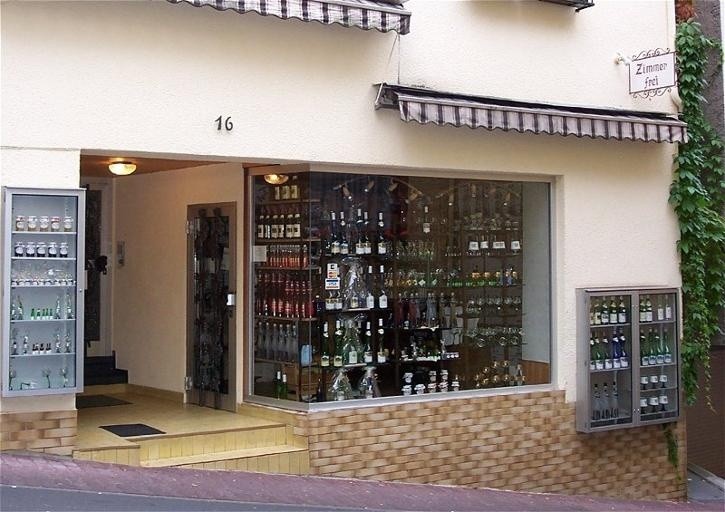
top-left (574, 285), bottom-right (684, 434)
top-left (0, 186), bottom-right (88, 399)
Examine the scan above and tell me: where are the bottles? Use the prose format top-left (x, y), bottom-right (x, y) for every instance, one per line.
top-left (363, 212), bottom-right (372, 254)
top-left (610, 296), bottom-right (618, 324)
top-left (415, 293), bottom-right (420, 327)
top-left (19, 279), bottom-right (24, 286)
top-left (44, 280), bottom-right (51, 285)
top-left (25, 279), bottom-right (31, 285)
top-left (264, 208), bottom-right (271, 239)
top-left (39, 343), bottom-right (44, 355)
top-left (67, 279), bottom-right (73, 285)
top-left (317, 378), bottom-right (322, 402)
top-left (14, 241), bottom-right (23, 256)
top-left (593, 381), bottom-right (618, 420)
top-left (281, 181), bottom-right (289, 199)
top-left (27, 216), bottom-right (36, 231)
top-left (39, 279), bottom-right (44, 285)
top-left (426, 293), bottom-right (434, 327)
top-left (263, 322), bottom-right (273, 359)
top-left (595, 337), bottom-right (603, 370)
top-left (63, 217), bottom-right (72, 231)
top-left (375, 319), bottom-right (386, 363)
top-left (443, 301), bottom-right (450, 328)
top-left (640, 330), bottom-right (649, 366)
top-left (37, 242), bottom-right (46, 257)
top-left (292, 173), bottom-right (300, 201)
top-left (398, 293), bottom-right (404, 328)
top-left (26, 242), bottom-right (35, 256)
top-left (36, 308), bottom-right (40, 320)
top-left (663, 328), bottom-right (671, 363)
top-left (255, 271), bottom-right (314, 318)
top-left (50, 216), bottom-right (59, 232)
top-left (42, 309), bottom-right (45, 320)
top-left (39, 216), bottom-right (49, 232)
top-left (285, 207), bottom-right (294, 239)
top-left (646, 294), bottom-right (652, 322)
top-left (377, 265), bottom-right (388, 309)
top-left (278, 324), bottom-right (285, 361)
top-left (590, 299), bottom-right (594, 326)
top-left (46, 308), bottom-right (48, 320)
top-left (271, 324), bottom-right (279, 360)
top-left (422, 206), bottom-right (431, 236)
top-left (601, 297), bottom-right (609, 324)
top-left (330, 213), bottom-right (340, 254)
top-left (355, 208), bottom-right (364, 254)
top-left (36, 343), bottom-right (38, 354)
top-left (375, 212), bottom-right (387, 255)
top-left (618, 295), bottom-right (627, 323)
top-left (432, 293), bottom-right (437, 327)
top-left (291, 325), bottom-right (297, 362)
top-left (655, 296), bottom-right (664, 321)
top-left (30, 308), bottom-right (35, 320)
top-left (648, 327), bottom-right (656, 365)
top-left (275, 183), bottom-right (280, 200)
top-left (271, 209), bottom-right (278, 239)
top-left (46, 343), bottom-right (49, 354)
top-left (293, 206), bottom-right (300, 238)
top-left (594, 297), bottom-right (601, 324)
top-left (16, 215), bottom-right (25, 231)
top-left (365, 266), bottom-right (375, 308)
top-left (639, 294), bottom-right (646, 323)
top-left (590, 338), bottom-right (595, 370)
top-left (280, 374), bottom-right (287, 399)
top-left (59, 243), bottom-right (68, 257)
top-left (320, 321), bottom-right (330, 366)
top-left (609, 330), bottom-right (620, 368)
top-left (32, 279), bottom-right (37, 285)
top-left (32, 344), bottom-right (35, 354)
top-left (338, 212), bottom-right (348, 254)
top-left (438, 293), bottom-right (446, 327)
top-left (340, 322), bottom-right (357, 364)
top-left (449, 292), bottom-right (457, 327)
top-left (60, 279), bottom-right (66, 286)
top-left (285, 324), bottom-right (292, 362)
top-left (619, 329), bottom-right (628, 368)
top-left (11, 279), bottom-right (17, 285)
top-left (275, 371), bottom-right (281, 399)
top-left (257, 208), bottom-right (265, 238)
top-left (664, 295), bottom-right (672, 320)
top-left (54, 279), bottom-right (60, 285)
top-left (256, 322), bottom-right (265, 358)
top-left (48, 242), bottom-right (57, 257)
top-left (602, 337), bottom-right (611, 367)
top-left (49, 309), bottom-right (53, 320)
top-left (409, 293), bottom-right (417, 327)
top-left (49, 342), bottom-right (52, 355)
top-left (278, 208), bottom-right (285, 238)
top-left (266, 244), bottom-right (307, 268)
top-left (654, 328), bottom-right (664, 364)
top-left (333, 321), bottom-right (341, 366)
top-left (363, 321), bottom-right (372, 363)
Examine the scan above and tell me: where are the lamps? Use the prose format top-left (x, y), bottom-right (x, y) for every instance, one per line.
top-left (263, 174), bottom-right (289, 185)
top-left (108, 157), bottom-right (137, 175)
